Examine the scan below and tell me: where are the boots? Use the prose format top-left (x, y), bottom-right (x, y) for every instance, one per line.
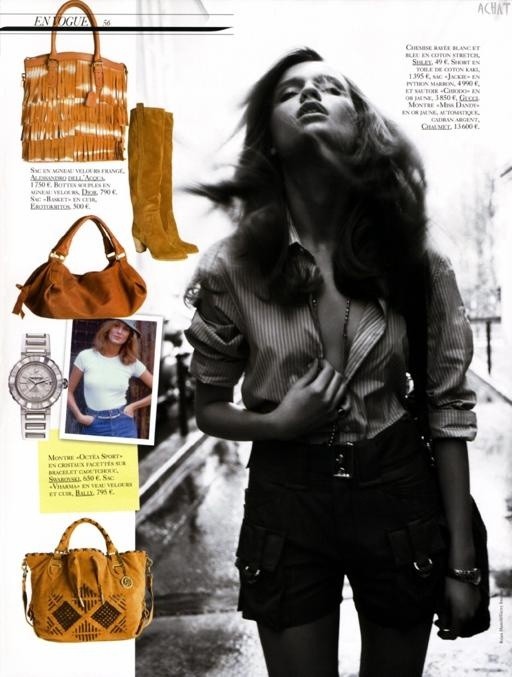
top-left (128, 101), bottom-right (200, 261)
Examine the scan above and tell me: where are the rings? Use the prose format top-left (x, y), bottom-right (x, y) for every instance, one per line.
top-left (335, 405), bottom-right (347, 422)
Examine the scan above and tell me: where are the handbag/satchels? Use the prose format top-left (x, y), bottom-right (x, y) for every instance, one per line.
top-left (436, 494), bottom-right (490, 638)
top-left (22, 517), bottom-right (154, 644)
top-left (12, 214), bottom-right (148, 320)
top-left (20, 0), bottom-right (129, 162)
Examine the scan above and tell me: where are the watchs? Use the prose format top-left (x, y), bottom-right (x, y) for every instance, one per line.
top-left (7, 329), bottom-right (70, 439)
top-left (444, 563), bottom-right (483, 589)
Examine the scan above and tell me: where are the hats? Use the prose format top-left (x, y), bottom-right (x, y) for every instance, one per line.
top-left (119, 319), bottom-right (143, 337)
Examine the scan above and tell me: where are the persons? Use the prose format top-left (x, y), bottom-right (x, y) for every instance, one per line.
top-left (187, 44), bottom-right (490, 677)
top-left (66, 318), bottom-right (154, 438)
